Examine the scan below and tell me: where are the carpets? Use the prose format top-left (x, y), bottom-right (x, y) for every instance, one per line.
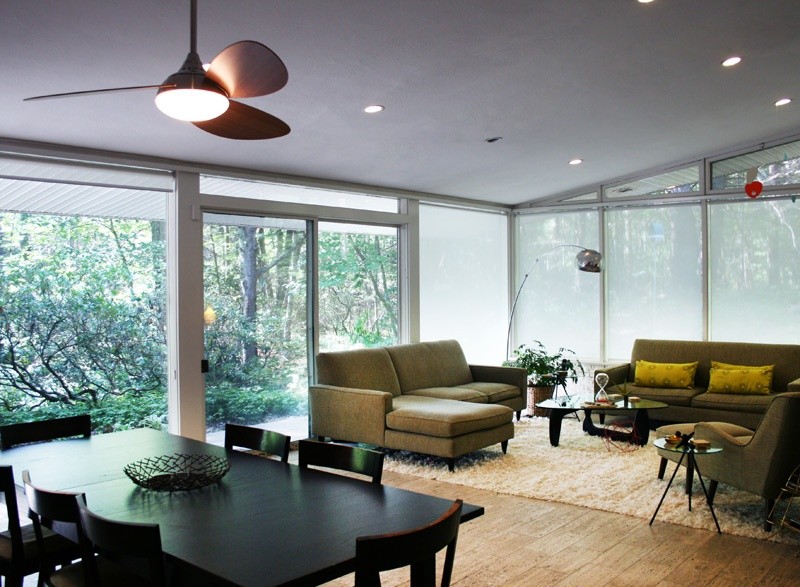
top-left (317, 413), bottom-right (800, 544)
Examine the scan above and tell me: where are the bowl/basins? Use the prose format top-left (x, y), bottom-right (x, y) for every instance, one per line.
top-left (124, 453), bottom-right (231, 490)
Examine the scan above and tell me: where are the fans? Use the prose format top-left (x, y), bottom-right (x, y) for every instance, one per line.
top-left (23, 1), bottom-right (292, 141)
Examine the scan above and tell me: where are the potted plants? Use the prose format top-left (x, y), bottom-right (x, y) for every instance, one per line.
top-left (502, 339), bottom-right (586, 416)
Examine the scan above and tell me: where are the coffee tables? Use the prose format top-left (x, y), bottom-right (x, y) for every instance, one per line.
top-left (535, 395), bottom-right (669, 447)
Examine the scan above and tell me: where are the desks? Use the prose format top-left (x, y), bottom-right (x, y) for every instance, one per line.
top-left (649, 437), bottom-right (725, 535)
top-left (0, 426), bottom-right (484, 587)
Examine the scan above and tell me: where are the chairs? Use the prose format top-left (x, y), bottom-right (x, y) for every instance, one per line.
top-left (0, 414), bottom-right (463, 587)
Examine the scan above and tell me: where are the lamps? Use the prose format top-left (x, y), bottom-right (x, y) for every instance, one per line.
top-left (154, 50), bottom-right (231, 124)
top-left (505, 244), bottom-right (602, 360)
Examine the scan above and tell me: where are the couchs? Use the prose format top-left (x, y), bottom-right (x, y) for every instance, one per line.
top-left (308, 337), bottom-right (528, 474)
top-left (593, 338), bottom-right (800, 431)
top-left (694, 389), bottom-right (800, 520)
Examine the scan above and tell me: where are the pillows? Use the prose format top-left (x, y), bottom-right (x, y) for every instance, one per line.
top-left (632, 359), bottom-right (775, 396)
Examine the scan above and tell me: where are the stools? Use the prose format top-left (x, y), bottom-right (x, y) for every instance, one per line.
top-left (637, 422), bottom-right (757, 480)
